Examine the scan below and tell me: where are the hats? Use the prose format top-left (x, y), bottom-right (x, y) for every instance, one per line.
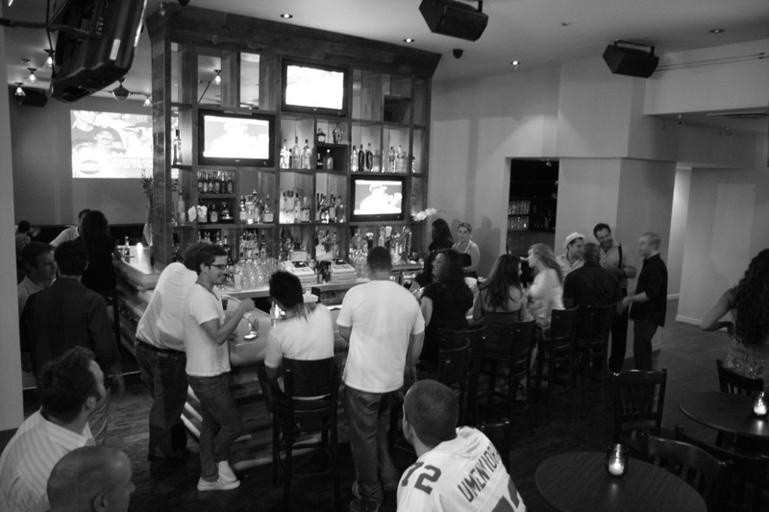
top-left (564, 231), bottom-right (585, 251)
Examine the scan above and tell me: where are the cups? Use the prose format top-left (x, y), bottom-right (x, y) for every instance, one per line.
top-left (248, 314), bottom-right (257, 333)
top-left (230, 257), bottom-right (276, 292)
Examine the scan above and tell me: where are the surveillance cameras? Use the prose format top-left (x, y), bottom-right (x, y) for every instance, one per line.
top-left (452, 47), bottom-right (464, 59)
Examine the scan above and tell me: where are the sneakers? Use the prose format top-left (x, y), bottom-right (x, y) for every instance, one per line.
top-left (352, 479), bottom-right (362, 500)
top-left (216, 459), bottom-right (239, 481)
top-left (197, 476), bottom-right (241, 491)
top-left (276, 427), bottom-right (301, 451)
top-left (383, 482), bottom-right (398, 491)
top-left (296, 416), bottom-right (325, 433)
top-left (172, 447), bottom-right (201, 465)
top-left (158, 469), bottom-right (197, 487)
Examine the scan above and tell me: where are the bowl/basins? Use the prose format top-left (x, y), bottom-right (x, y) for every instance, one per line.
top-left (384, 94), bottom-right (412, 122)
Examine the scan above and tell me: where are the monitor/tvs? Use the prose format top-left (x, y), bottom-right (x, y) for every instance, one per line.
top-left (52, 0), bottom-right (148, 103)
top-left (281, 59), bottom-right (348, 116)
top-left (350, 175), bottom-right (406, 221)
top-left (199, 111), bottom-right (276, 167)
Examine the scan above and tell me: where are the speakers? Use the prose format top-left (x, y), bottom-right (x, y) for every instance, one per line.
top-left (603, 45), bottom-right (660, 78)
top-left (13, 88), bottom-right (48, 107)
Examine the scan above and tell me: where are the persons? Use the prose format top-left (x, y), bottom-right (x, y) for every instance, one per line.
top-left (405, 216), bottom-right (670, 405)
top-left (132, 239), bottom-right (213, 474)
top-left (392, 376), bottom-right (525, 512)
top-left (183, 242), bottom-right (259, 497)
top-left (44, 440), bottom-right (139, 511)
top-left (695, 248), bottom-right (768, 396)
top-left (1, 341), bottom-right (110, 512)
top-left (254, 267), bottom-right (339, 416)
top-left (14, 205), bottom-right (118, 409)
top-left (332, 243), bottom-right (426, 509)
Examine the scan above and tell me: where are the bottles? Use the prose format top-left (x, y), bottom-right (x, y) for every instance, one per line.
top-left (607, 442), bottom-right (627, 476)
top-left (172, 129), bottom-right (185, 166)
top-left (239, 191), bottom-right (275, 260)
top-left (317, 148), bottom-right (334, 171)
top-left (351, 142), bottom-right (375, 172)
top-left (317, 127), bottom-right (326, 143)
top-left (281, 135), bottom-right (310, 170)
top-left (333, 122), bottom-right (343, 145)
top-left (278, 238), bottom-right (294, 261)
top-left (315, 230), bottom-right (339, 262)
top-left (280, 187), bottom-right (345, 225)
top-left (124, 236), bottom-right (130, 256)
top-left (176, 188), bottom-right (186, 225)
top-left (752, 391), bottom-right (769, 416)
top-left (350, 225), bottom-right (412, 264)
top-left (197, 169), bottom-right (234, 264)
top-left (387, 146), bottom-right (416, 173)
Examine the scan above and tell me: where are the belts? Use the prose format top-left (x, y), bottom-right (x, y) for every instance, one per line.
top-left (133, 338), bottom-right (187, 358)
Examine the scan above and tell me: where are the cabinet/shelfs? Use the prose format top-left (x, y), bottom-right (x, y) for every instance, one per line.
top-left (145, 1), bottom-right (444, 269)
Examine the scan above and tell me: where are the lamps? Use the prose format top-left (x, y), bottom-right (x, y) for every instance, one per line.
top-left (601, 38), bottom-right (661, 80)
top-left (43, 47), bottom-right (57, 67)
top-left (113, 73), bottom-right (132, 104)
top-left (27, 66), bottom-right (37, 81)
top-left (14, 81), bottom-right (26, 97)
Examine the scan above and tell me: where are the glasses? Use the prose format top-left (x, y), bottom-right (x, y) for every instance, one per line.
top-left (205, 264), bottom-right (230, 269)
